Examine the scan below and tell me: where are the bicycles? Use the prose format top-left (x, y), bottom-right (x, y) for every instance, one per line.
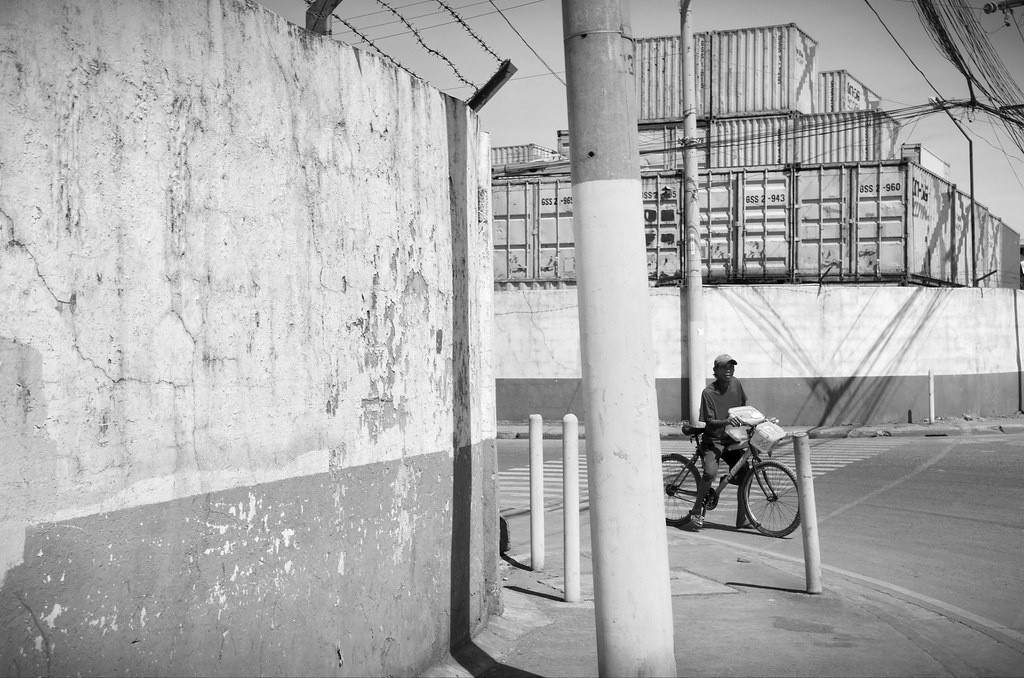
top-left (661, 419), bottom-right (801, 537)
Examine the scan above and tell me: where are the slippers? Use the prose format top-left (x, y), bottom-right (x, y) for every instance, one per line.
top-left (736, 523), bottom-right (762, 529)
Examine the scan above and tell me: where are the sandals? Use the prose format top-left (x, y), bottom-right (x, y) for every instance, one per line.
top-left (689, 510), bottom-right (703, 528)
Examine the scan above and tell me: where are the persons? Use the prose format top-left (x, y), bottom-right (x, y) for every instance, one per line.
top-left (688, 354), bottom-right (776, 530)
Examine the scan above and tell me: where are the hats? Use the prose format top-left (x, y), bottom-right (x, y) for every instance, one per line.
top-left (713, 354), bottom-right (738, 368)
